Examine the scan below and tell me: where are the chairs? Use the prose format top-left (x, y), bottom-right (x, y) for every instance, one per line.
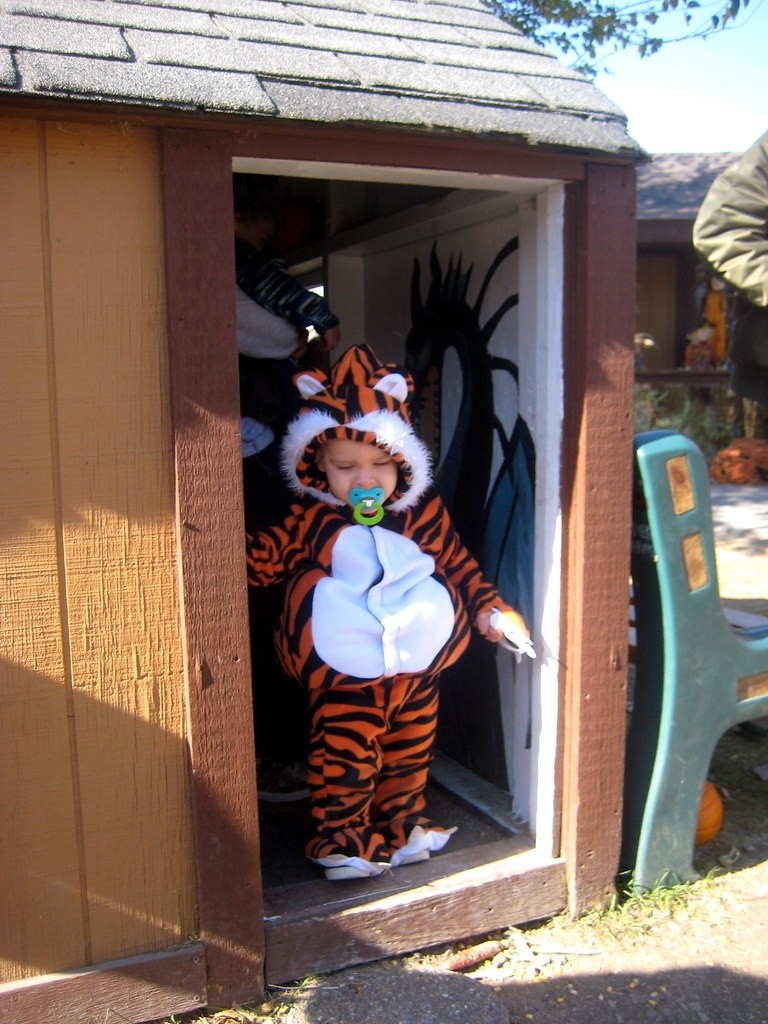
top-left (626, 431), bottom-right (768, 894)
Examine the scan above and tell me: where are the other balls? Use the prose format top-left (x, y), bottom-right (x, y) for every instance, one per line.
top-left (694, 780), bottom-right (724, 846)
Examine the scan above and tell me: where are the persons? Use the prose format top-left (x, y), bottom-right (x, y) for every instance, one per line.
top-left (234, 170), bottom-right (342, 462)
top-left (676, 317), bottom-right (724, 435)
top-left (690, 125), bottom-right (768, 309)
top-left (241, 341), bottom-right (536, 882)
